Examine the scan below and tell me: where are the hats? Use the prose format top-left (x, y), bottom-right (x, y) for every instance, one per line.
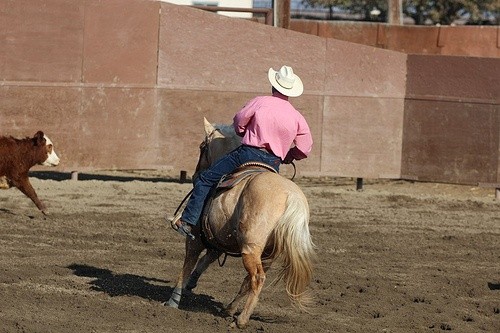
top-left (267, 65), bottom-right (303, 98)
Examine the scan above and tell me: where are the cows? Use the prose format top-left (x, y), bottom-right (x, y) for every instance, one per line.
top-left (0, 128), bottom-right (61, 219)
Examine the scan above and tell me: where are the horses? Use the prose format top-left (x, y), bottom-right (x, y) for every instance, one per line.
top-left (164, 117), bottom-right (321, 333)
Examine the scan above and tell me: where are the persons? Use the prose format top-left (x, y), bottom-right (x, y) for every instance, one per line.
top-left (165, 65), bottom-right (314, 238)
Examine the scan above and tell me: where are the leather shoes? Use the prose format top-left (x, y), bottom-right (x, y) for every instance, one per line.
top-left (166, 213), bottom-right (193, 235)
